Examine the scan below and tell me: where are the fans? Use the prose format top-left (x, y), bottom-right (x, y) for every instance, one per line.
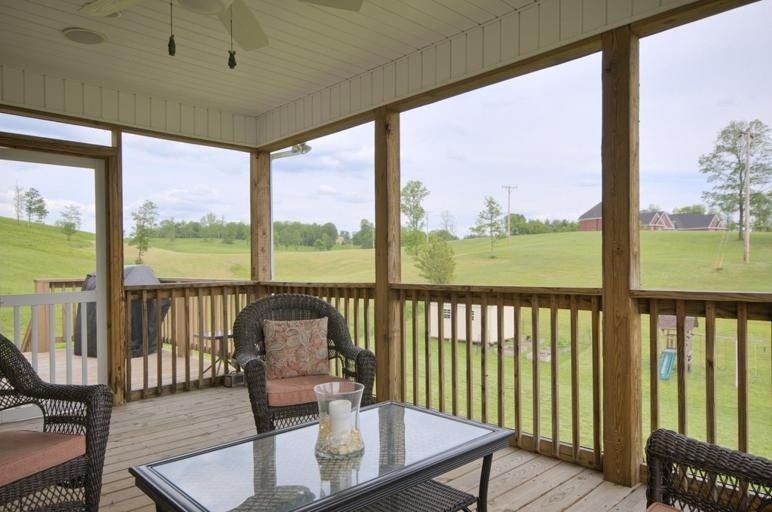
top-left (76, 0), bottom-right (365, 52)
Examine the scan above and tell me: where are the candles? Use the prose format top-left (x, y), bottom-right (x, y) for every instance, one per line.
top-left (328, 399), bottom-right (353, 436)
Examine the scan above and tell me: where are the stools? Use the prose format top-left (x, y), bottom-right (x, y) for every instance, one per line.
top-left (193, 329), bottom-right (241, 377)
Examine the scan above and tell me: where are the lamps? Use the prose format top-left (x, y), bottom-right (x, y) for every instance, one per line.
top-left (299, 141), bottom-right (313, 155)
top-left (291, 144), bottom-right (300, 153)
top-left (168, 0), bottom-right (237, 70)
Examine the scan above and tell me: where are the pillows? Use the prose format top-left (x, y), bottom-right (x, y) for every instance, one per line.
top-left (259, 316), bottom-right (330, 381)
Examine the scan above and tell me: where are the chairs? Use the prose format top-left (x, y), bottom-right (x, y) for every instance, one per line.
top-left (235, 293), bottom-right (379, 435)
top-left (643, 429), bottom-right (771, 512)
top-left (0, 332), bottom-right (115, 512)
